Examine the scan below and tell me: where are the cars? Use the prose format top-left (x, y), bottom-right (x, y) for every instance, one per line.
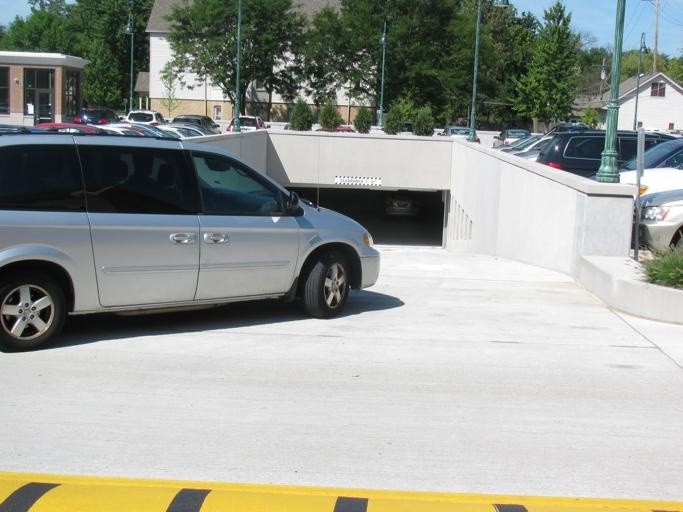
top-left (384, 194), bottom-right (423, 216)
top-left (492, 124), bottom-right (683, 232)
top-left (437, 126), bottom-right (479, 142)
top-left (1, 107), bottom-right (271, 138)
top-left (316, 127), bottom-right (354, 131)
top-left (395, 121), bottom-right (416, 135)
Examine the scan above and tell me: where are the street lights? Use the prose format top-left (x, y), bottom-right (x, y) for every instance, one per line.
top-left (121, 12), bottom-right (135, 110)
top-left (378, 20), bottom-right (387, 127)
top-left (468, 1), bottom-right (507, 142)
top-left (634, 33), bottom-right (647, 129)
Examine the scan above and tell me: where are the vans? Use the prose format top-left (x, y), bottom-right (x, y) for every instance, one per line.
top-left (1, 134), bottom-right (379, 331)
